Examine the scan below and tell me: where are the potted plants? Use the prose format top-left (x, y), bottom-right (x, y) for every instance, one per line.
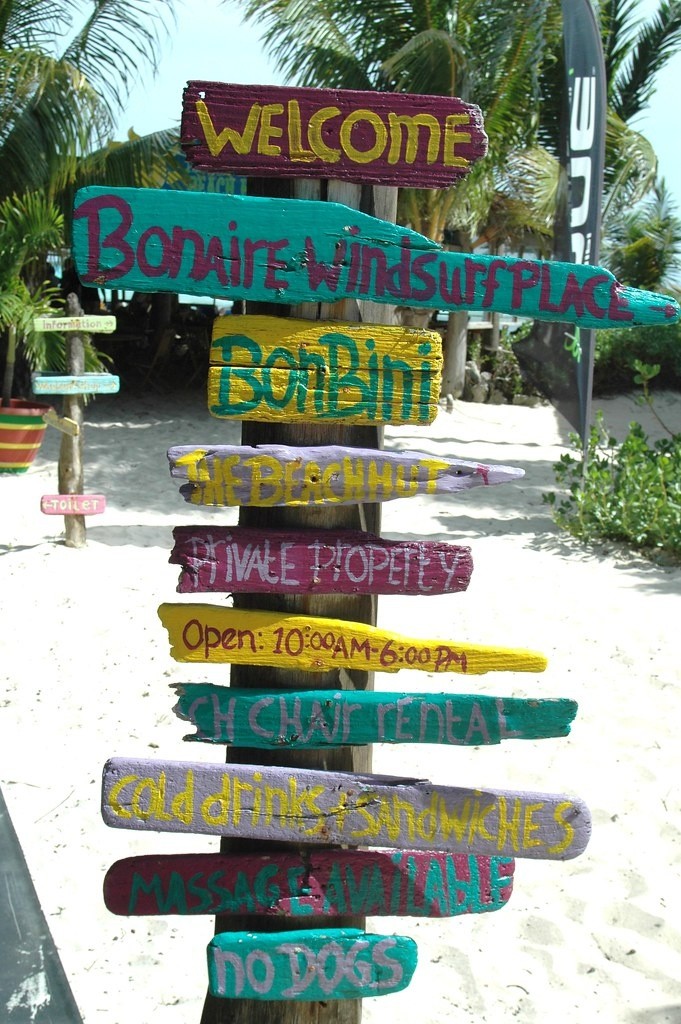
top-left (0, 185), bottom-right (113, 477)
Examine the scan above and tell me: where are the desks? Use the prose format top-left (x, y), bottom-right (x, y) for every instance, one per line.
top-left (95, 333), bottom-right (142, 394)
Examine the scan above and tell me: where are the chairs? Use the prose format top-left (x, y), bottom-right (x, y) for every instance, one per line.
top-left (126, 327), bottom-right (176, 398)
